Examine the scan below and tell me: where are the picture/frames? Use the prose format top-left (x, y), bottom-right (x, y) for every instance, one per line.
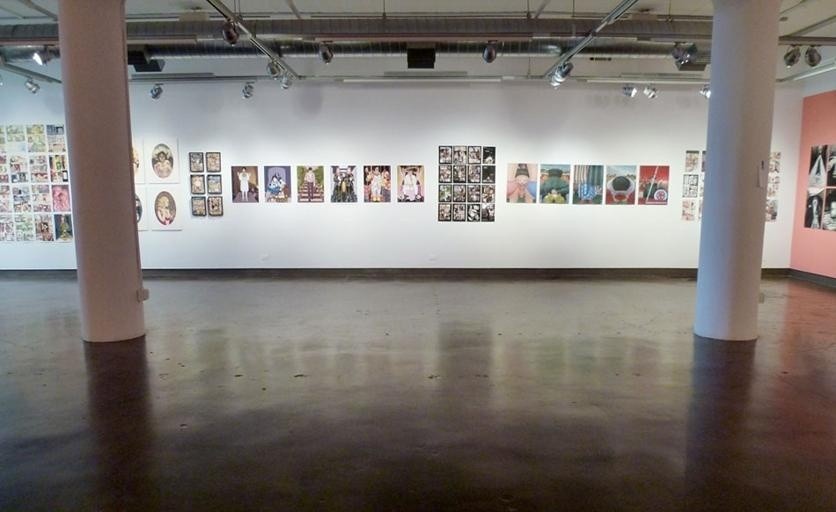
top-left (436, 143), bottom-right (496, 225)
top-left (185, 150), bottom-right (226, 218)
top-left (130, 133), bottom-right (185, 234)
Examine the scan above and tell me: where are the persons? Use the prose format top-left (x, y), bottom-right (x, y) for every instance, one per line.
top-left (331, 167), bottom-right (357, 202)
top-left (152, 152), bottom-right (172, 177)
top-left (269, 176), bottom-right (288, 199)
top-left (304, 167), bottom-right (315, 202)
top-left (156, 196), bottom-right (173, 224)
top-left (237, 168), bottom-right (252, 201)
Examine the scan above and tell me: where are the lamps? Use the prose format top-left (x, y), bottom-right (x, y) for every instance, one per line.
top-left (699, 87), bottom-right (710, 98)
top-left (642, 87), bottom-right (659, 98)
top-left (34, 48), bottom-right (55, 64)
top-left (280, 73), bottom-right (294, 90)
top-left (484, 46), bottom-right (497, 63)
top-left (319, 45), bottom-right (333, 63)
top-left (266, 62), bottom-right (281, 79)
top-left (805, 48), bottom-right (822, 67)
top-left (672, 44), bottom-right (698, 65)
top-left (25, 81), bottom-right (40, 94)
top-left (243, 83), bottom-right (253, 99)
top-left (622, 87), bottom-right (636, 97)
top-left (784, 49), bottom-right (800, 68)
top-left (222, 23), bottom-right (238, 44)
top-left (151, 83), bottom-right (163, 98)
top-left (550, 63), bottom-right (574, 88)
